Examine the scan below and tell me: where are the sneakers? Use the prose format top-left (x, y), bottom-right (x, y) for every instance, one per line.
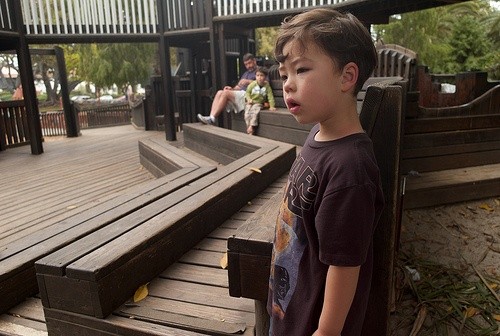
top-left (197, 113), bottom-right (216, 126)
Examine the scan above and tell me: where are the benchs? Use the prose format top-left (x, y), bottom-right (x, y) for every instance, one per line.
top-left (223, 76), bottom-right (409, 336)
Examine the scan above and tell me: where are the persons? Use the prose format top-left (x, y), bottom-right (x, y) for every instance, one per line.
top-left (266, 7), bottom-right (385, 336)
top-left (197, 53), bottom-right (269, 125)
top-left (243, 68), bottom-right (277, 134)
top-left (12, 83), bottom-right (24, 100)
